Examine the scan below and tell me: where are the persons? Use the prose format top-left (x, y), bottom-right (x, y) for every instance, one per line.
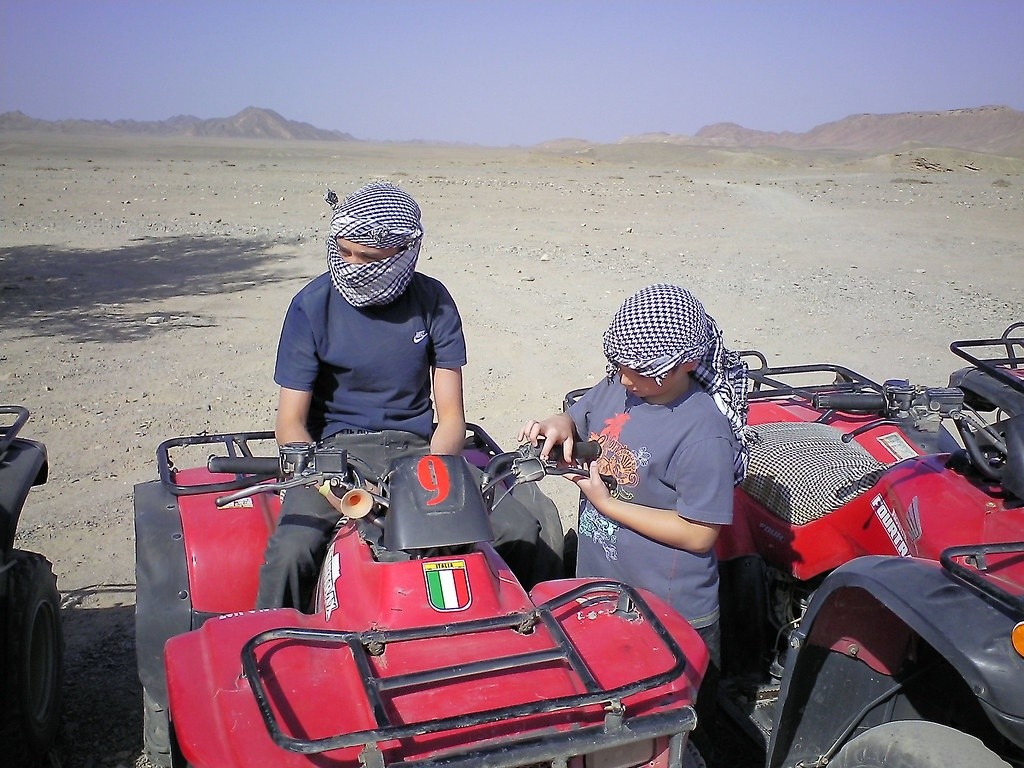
top-left (517, 285), bottom-right (749, 718)
top-left (252, 181), bottom-right (541, 614)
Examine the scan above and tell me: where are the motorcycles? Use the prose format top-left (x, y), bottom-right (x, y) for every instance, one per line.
top-left (566, 324), bottom-right (1024, 768)
top-left (1, 405), bottom-right (62, 764)
top-left (129, 420), bottom-right (706, 768)
